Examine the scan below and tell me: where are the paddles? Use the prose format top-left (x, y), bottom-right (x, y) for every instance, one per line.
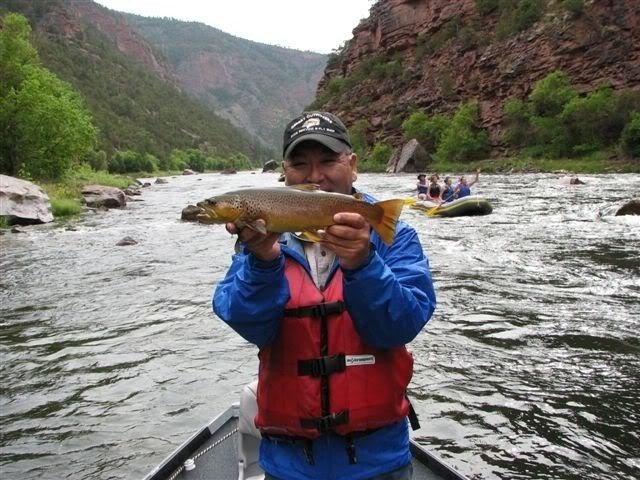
top-left (403, 198), bottom-right (432, 203)
top-left (425, 190), bottom-right (461, 216)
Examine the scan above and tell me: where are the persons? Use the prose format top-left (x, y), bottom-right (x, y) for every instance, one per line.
top-left (427, 174), bottom-right (441, 201)
top-left (438, 176), bottom-right (458, 204)
top-left (417, 173), bottom-right (429, 201)
top-left (453, 168), bottom-right (480, 199)
top-left (212, 112), bottom-right (436, 479)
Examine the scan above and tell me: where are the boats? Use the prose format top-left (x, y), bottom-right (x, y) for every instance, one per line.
top-left (410, 195), bottom-right (493, 218)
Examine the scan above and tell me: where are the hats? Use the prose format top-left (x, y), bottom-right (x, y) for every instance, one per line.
top-left (418, 174), bottom-right (426, 179)
top-left (282, 111), bottom-right (351, 159)
